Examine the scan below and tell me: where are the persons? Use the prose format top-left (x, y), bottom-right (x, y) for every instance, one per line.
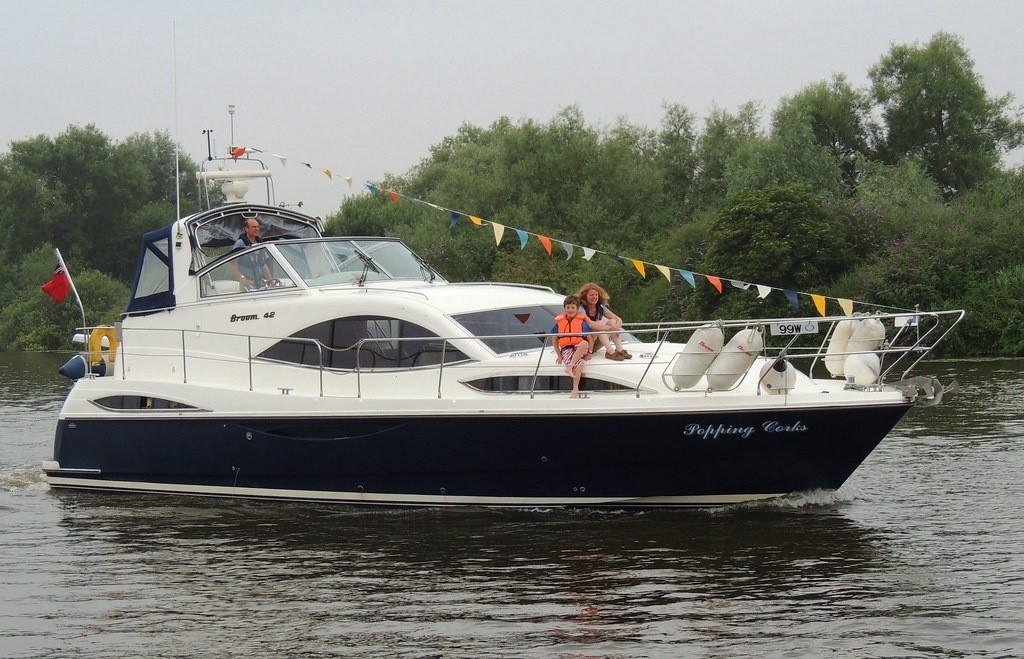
top-left (229, 219), bottom-right (274, 292)
top-left (578, 283), bottom-right (632, 361)
top-left (550, 296), bottom-right (594, 399)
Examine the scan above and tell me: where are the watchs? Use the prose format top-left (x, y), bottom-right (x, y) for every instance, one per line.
top-left (239, 275), bottom-right (246, 282)
top-left (588, 350), bottom-right (593, 354)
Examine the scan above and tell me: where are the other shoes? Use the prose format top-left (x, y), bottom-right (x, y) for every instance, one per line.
top-left (605, 351), bottom-right (624, 361)
top-left (616, 349), bottom-right (632, 359)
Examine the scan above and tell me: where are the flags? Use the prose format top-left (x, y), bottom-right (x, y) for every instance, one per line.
top-left (42, 262), bottom-right (71, 302)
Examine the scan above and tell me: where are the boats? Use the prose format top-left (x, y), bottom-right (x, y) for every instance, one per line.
top-left (40, 100), bottom-right (969, 514)
